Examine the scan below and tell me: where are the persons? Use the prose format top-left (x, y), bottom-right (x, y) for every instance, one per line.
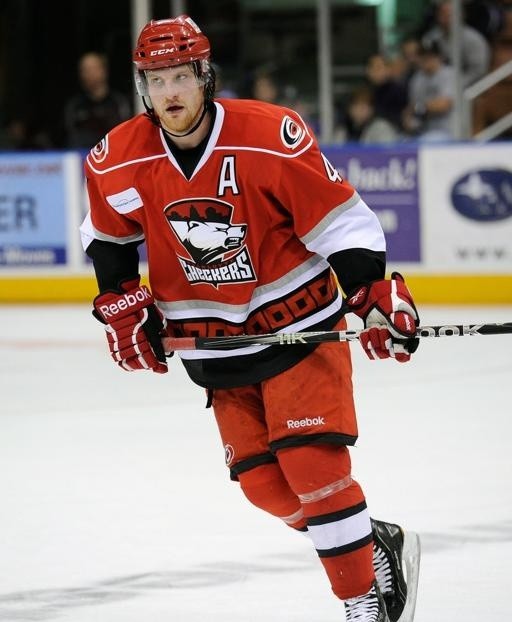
top-left (1, 1), bottom-right (511, 149)
top-left (80, 18), bottom-right (423, 622)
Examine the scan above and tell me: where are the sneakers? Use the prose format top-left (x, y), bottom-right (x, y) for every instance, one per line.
top-left (344, 578), bottom-right (386, 622)
top-left (369, 516), bottom-right (407, 622)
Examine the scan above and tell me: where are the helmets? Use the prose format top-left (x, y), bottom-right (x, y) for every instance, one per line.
top-left (133, 14), bottom-right (212, 96)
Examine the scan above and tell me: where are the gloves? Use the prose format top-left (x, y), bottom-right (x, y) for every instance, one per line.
top-left (94, 275), bottom-right (174, 373)
top-left (345, 272), bottom-right (419, 363)
